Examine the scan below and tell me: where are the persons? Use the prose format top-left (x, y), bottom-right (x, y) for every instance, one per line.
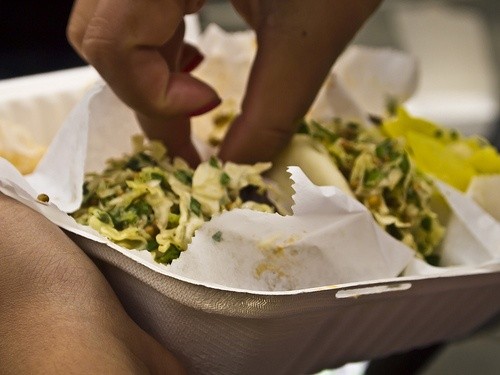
top-left (0, 1), bottom-right (500, 375)
